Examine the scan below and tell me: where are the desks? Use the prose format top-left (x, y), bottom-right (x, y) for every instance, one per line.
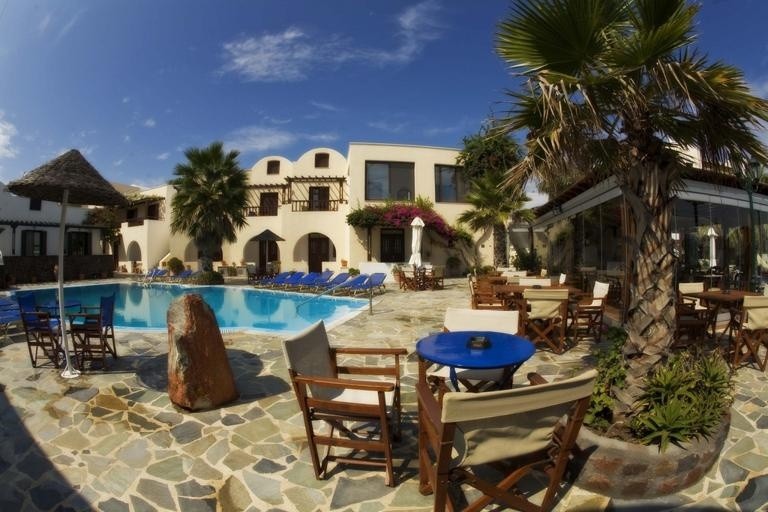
top-left (3, 254), bottom-right (61, 282)
top-left (63, 252), bottom-right (118, 279)
top-left (415, 330), bottom-right (536, 394)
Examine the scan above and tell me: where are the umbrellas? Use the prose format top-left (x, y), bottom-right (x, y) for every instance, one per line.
top-left (707, 226), bottom-right (719, 271)
top-left (408, 217), bottom-right (425, 276)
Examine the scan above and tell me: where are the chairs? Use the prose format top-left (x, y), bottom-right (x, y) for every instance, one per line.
top-left (413, 307), bottom-right (522, 393)
top-left (143, 266), bottom-right (388, 297)
top-left (395, 261), bottom-right (446, 293)
top-left (413, 364), bottom-right (601, 512)
top-left (280, 317), bottom-right (412, 489)
top-left (463, 257), bottom-right (767, 373)
top-left (0, 295), bottom-right (120, 372)
